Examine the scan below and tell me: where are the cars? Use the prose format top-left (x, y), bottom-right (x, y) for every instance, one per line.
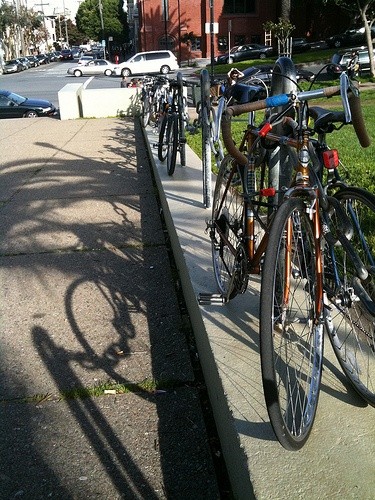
top-left (353, 49), bottom-right (375, 76)
top-left (112, 49), bottom-right (180, 79)
top-left (274, 23), bottom-right (375, 56)
top-left (0, 89), bottom-right (65, 116)
top-left (67, 58), bottom-right (118, 78)
top-left (214, 43), bottom-right (275, 66)
top-left (2, 40), bottom-right (110, 75)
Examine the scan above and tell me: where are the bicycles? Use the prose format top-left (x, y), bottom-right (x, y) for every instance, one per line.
top-left (125, 62), bottom-right (375, 452)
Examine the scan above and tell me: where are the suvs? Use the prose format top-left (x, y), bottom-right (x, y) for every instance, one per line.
top-left (327, 48), bottom-right (357, 78)
